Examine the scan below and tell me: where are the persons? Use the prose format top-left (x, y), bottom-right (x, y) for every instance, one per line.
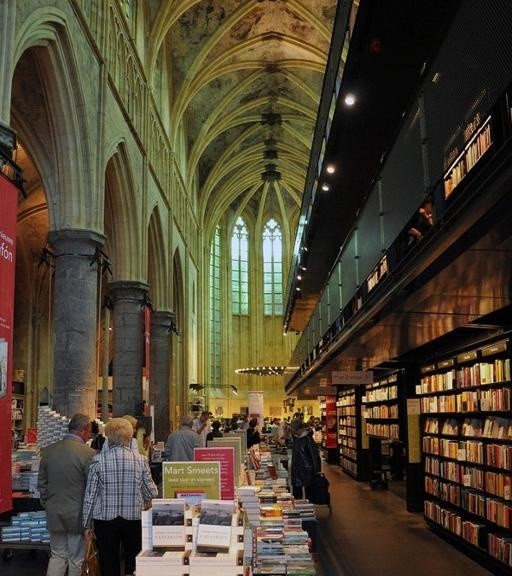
top-left (36, 413), bottom-right (99, 576)
top-left (291, 422), bottom-right (321, 499)
top-left (87, 421), bottom-right (105, 449)
top-left (102, 414), bottom-right (140, 454)
top-left (192, 411), bottom-right (210, 446)
top-left (225, 406), bottom-right (324, 450)
top-left (164, 415), bottom-right (203, 461)
top-left (79, 417), bottom-right (159, 576)
top-left (205, 419), bottom-right (223, 446)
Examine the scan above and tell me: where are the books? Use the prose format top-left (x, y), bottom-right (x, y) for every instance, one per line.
top-left (421, 358), bottom-right (512, 567)
top-left (336, 395), bottom-right (356, 474)
top-left (364, 387), bottom-right (399, 455)
top-left (1, 404), bottom-right (71, 544)
top-left (367, 257), bottom-right (388, 291)
top-left (443, 123), bottom-right (492, 198)
top-left (133, 428), bottom-right (319, 575)
top-left (321, 411), bottom-right (336, 447)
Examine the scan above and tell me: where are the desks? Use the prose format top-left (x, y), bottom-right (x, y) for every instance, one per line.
top-left (12, 491), bottom-right (41, 516)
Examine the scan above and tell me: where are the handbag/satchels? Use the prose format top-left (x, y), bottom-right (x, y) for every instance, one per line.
top-left (305, 472), bottom-right (331, 506)
top-left (79, 551), bottom-right (102, 576)
